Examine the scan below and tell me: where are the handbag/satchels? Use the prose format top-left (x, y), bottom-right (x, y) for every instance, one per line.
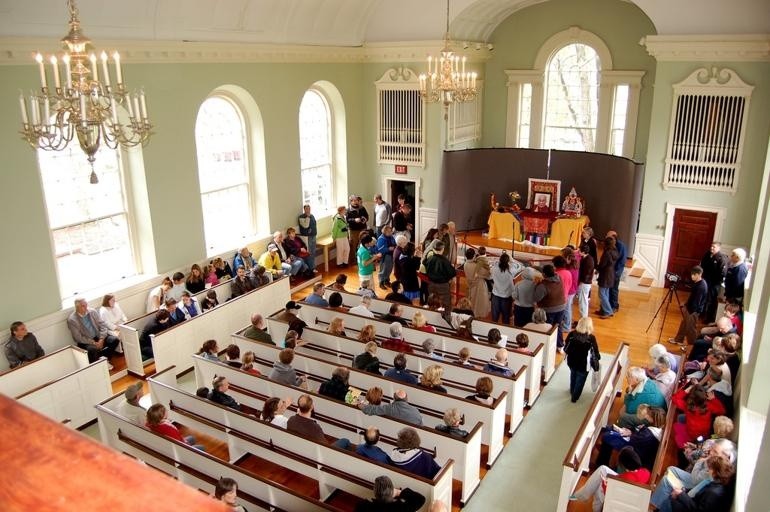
top-left (590, 355), bottom-right (599, 371)
top-left (604, 431), bottom-right (625, 450)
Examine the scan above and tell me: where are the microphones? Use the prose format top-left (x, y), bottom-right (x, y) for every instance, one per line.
top-left (468, 216), bottom-right (472, 222)
top-left (570, 230), bottom-right (574, 237)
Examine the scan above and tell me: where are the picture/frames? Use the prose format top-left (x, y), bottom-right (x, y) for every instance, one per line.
top-left (532, 192), bottom-right (551, 214)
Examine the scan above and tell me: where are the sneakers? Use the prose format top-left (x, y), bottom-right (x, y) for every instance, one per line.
top-left (595, 308), bottom-right (618, 318)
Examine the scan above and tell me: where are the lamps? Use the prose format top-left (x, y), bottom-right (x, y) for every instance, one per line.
top-left (418, 1), bottom-right (478, 122)
top-left (17, 1), bottom-right (154, 185)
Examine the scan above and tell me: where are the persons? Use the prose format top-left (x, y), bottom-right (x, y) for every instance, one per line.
top-left (4, 321), bottom-right (45, 369)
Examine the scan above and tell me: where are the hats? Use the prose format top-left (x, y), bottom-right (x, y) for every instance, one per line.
top-left (268, 244), bottom-right (279, 252)
top-left (125, 381), bottom-right (143, 399)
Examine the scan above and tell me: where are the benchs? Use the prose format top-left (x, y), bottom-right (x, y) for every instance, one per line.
top-left (0, 227), bottom-right (558, 510)
top-left (558, 342), bottom-right (735, 512)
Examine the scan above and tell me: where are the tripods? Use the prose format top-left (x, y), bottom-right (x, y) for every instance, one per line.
top-left (646, 286), bottom-right (686, 343)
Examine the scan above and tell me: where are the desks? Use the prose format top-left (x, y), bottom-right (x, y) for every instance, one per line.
top-left (485, 208), bottom-right (593, 251)
